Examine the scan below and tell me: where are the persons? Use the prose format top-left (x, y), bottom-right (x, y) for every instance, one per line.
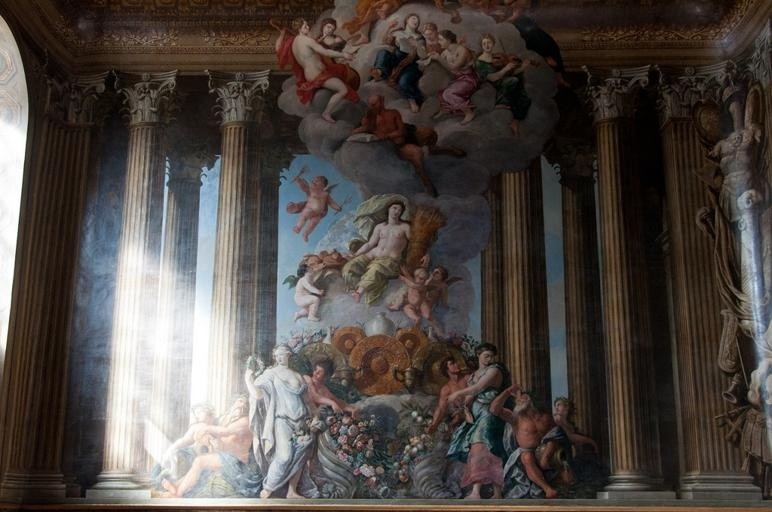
top-left (488, 383), bottom-right (578, 497)
top-left (300, 357), bottom-right (360, 422)
top-left (291, 175), bottom-right (342, 243)
top-left (399, 265), bottom-right (434, 323)
top-left (353, 94), bottom-right (467, 200)
top-left (163, 393), bottom-right (252, 495)
top-left (552, 391), bottom-right (599, 458)
top-left (290, 260), bottom-right (331, 327)
top-left (245, 341), bottom-right (314, 500)
top-left (164, 402), bottom-right (219, 488)
top-left (448, 341), bottom-right (511, 498)
top-left (427, 356), bottom-right (469, 435)
top-left (340, 199), bottom-right (432, 309)
top-left (427, 263), bottom-right (451, 308)
